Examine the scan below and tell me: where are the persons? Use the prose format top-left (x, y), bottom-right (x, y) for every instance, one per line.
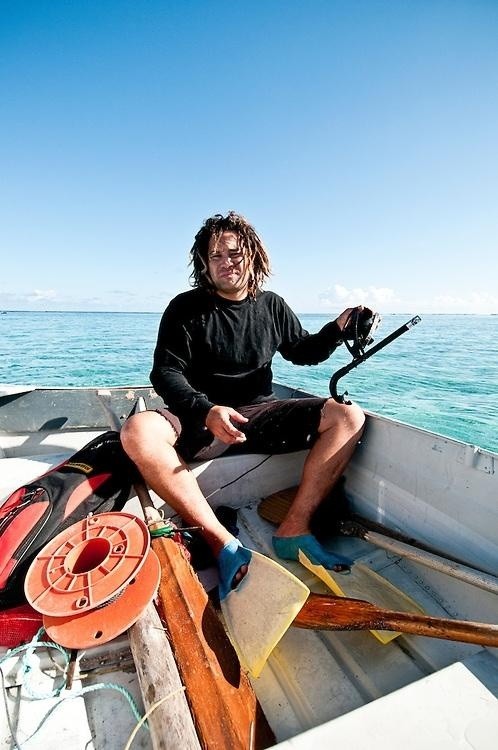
top-left (117, 210), bottom-right (374, 597)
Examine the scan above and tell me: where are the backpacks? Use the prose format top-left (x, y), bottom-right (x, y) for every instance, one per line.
top-left (0, 429), bottom-right (137, 610)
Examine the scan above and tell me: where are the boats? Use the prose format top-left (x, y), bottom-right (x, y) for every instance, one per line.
top-left (1, 380), bottom-right (498, 748)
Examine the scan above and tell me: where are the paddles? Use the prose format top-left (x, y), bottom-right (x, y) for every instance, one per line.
top-left (291, 592), bottom-right (497, 647)
top-left (257, 485), bottom-right (498, 596)
